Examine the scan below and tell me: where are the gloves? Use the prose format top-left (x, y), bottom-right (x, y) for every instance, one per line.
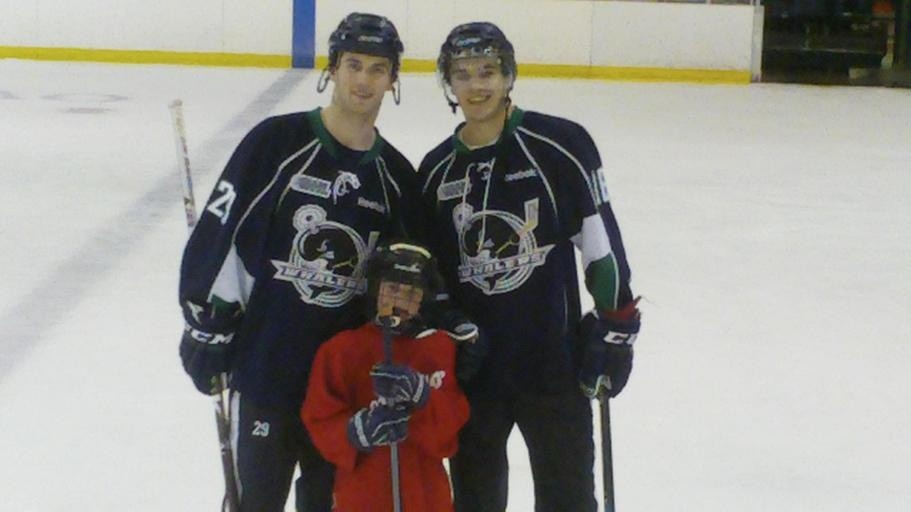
top-left (347, 402), bottom-right (411, 450)
top-left (368, 361), bottom-right (431, 412)
top-left (578, 310), bottom-right (641, 401)
top-left (178, 327), bottom-right (238, 398)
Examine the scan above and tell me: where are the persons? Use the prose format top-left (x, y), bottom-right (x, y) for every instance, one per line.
top-left (177, 11), bottom-right (495, 512)
top-left (299, 238), bottom-right (472, 510)
top-left (415, 18), bottom-right (641, 510)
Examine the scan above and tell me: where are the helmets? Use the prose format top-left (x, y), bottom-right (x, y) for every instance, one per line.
top-left (365, 243), bottom-right (440, 315)
top-left (327, 12), bottom-right (403, 78)
top-left (438, 22), bottom-right (516, 80)
